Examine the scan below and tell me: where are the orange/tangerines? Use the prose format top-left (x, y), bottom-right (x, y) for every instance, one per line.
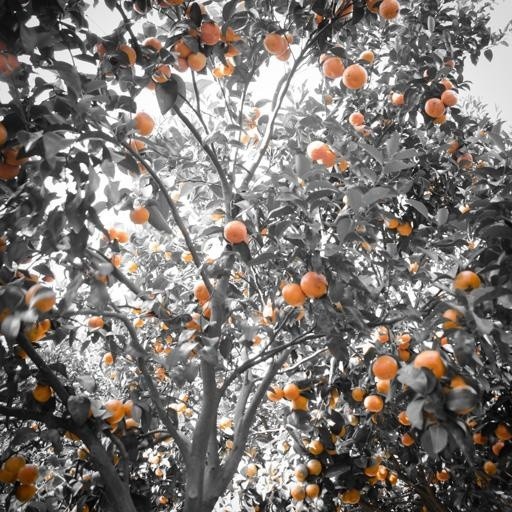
top-left (0, 0), bottom-right (512, 512)
